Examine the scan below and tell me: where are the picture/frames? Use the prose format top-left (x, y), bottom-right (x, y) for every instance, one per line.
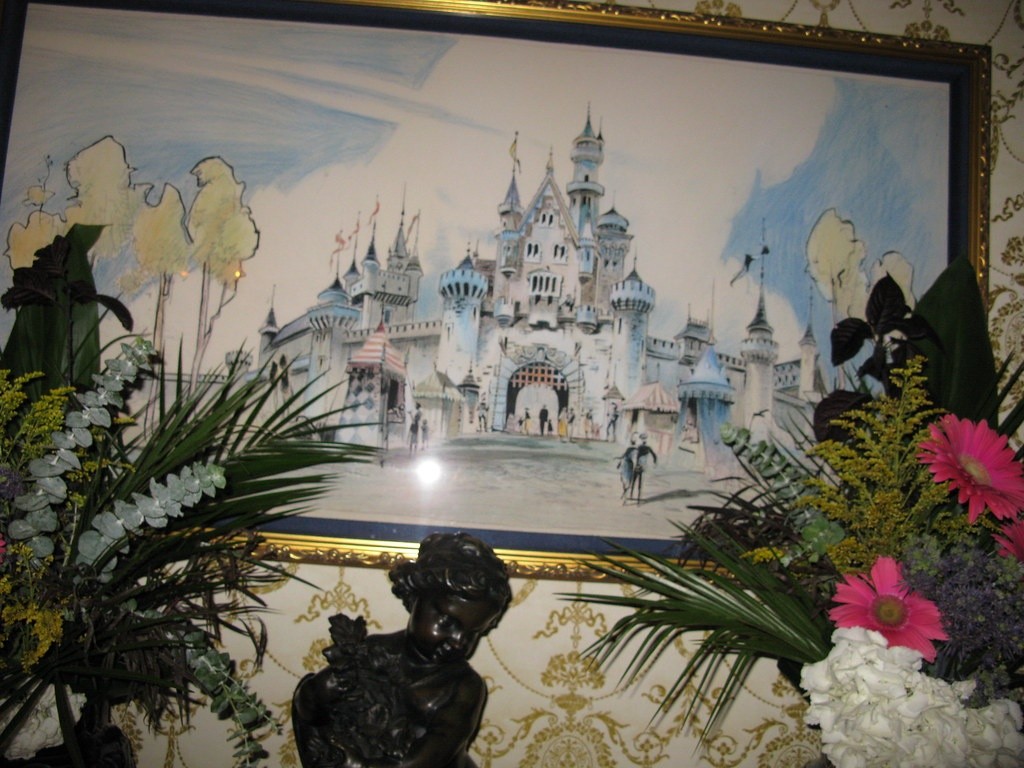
top-left (0, 0), bottom-right (992, 585)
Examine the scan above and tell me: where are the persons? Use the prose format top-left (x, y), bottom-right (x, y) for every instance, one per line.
top-left (291, 535), bottom-right (513, 768)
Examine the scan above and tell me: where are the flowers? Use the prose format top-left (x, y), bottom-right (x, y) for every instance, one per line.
top-left (558, 258), bottom-right (1024, 768)
top-left (0, 226), bottom-right (396, 768)
